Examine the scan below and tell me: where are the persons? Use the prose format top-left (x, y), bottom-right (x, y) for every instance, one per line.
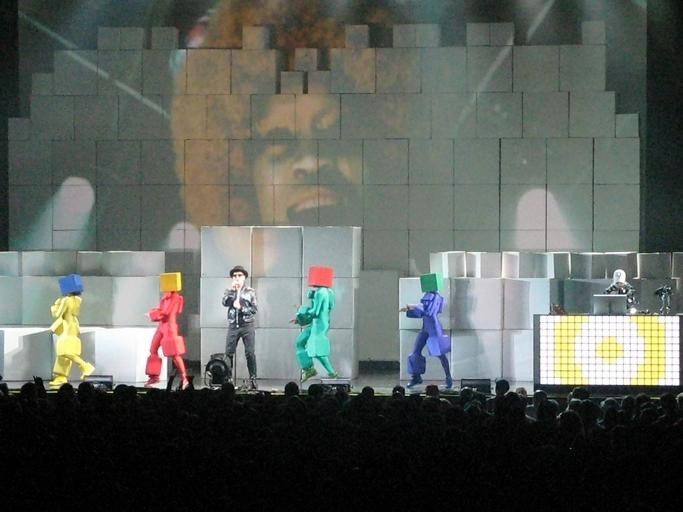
top-left (47, 274), bottom-right (97, 388)
top-left (399, 273), bottom-right (455, 389)
top-left (0, 376), bottom-right (683, 512)
top-left (142, 271), bottom-right (190, 391)
top-left (289, 266), bottom-right (339, 383)
top-left (602, 269), bottom-right (640, 314)
top-left (220, 266), bottom-right (259, 390)
top-left (161, 0), bottom-right (458, 268)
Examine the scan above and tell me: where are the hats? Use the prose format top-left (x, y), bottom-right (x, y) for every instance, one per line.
top-left (613, 270), bottom-right (626, 284)
top-left (307, 266), bottom-right (333, 287)
top-left (419, 273), bottom-right (443, 291)
top-left (160, 273), bottom-right (182, 291)
top-left (230, 265), bottom-right (248, 277)
top-left (59, 275), bottom-right (83, 294)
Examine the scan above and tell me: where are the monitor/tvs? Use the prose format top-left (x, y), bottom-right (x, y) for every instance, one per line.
top-left (593, 294), bottom-right (627, 316)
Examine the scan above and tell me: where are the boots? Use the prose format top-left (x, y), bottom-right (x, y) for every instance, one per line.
top-left (48, 375), bottom-right (68, 385)
top-left (145, 376), bottom-right (159, 386)
top-left (329, 373), bottom-right (339, 380)
top-left (180, 378), bottom-right (191, 390)
top-left (80, 363), bottom-right (95, 379)
top-left (301, 369), bottom-right (317, 382)
top-left (407, 374), bottom-right (423, 387)
top-left (445, 377), bottom-right (456, 389)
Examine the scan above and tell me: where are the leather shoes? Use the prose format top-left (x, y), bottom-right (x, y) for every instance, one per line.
top-left (251, 381), bottom-right (257, 389)
